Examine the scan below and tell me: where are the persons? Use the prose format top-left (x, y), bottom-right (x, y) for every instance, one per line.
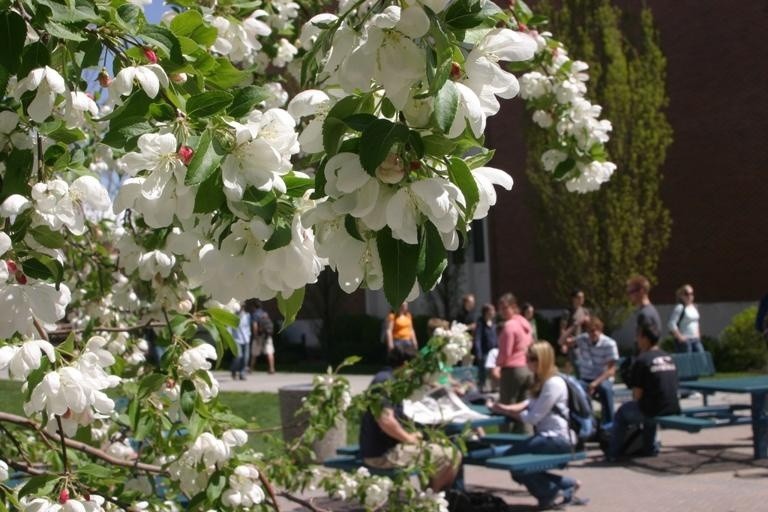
top-left (244, 300), bottom-right (275, 374)
top-left (227, 303), bottom-right (253, 381)
top-left (140, 326), bottom-right (168, 375)
top-left (666, 283), bottom-right (702, 401)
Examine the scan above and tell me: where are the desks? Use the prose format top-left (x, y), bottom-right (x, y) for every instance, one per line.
top-left (679, 376), bottom-right (767, 460)
top-left (416, 405), bottom-right (508, 511)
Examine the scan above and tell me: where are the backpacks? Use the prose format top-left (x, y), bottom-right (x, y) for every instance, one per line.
top-left (600, 398), bottom-right (647, 459)
top-left (538, 372), bottom-right (594, 442)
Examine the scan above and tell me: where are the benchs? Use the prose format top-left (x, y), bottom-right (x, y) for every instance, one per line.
top-left (593, 381), bottom-right (632, 403)
top-left (653, 403), bottom-right (752, 432)
top-left (325, 434), bottom-right (588, 471)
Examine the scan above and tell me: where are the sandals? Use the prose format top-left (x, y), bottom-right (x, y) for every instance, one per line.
top-left (539, 480), bottom-right (593, 510)
